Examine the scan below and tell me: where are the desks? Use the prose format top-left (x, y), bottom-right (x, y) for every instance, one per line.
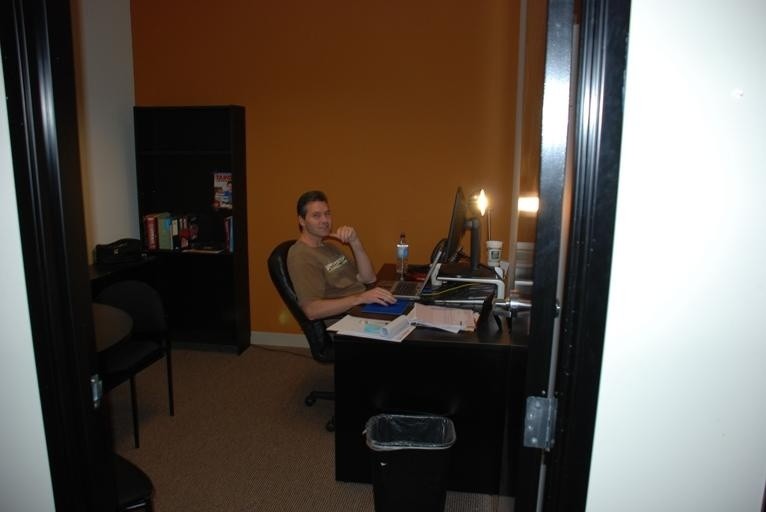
top-left (324, 263), bottom-right (528, 497)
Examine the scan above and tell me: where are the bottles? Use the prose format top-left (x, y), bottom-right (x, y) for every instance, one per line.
top-left (394, 231), bottom-right (410, 276)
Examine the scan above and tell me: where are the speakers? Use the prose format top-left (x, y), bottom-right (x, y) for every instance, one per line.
top-left (430, 238), bottom-right (447, 262)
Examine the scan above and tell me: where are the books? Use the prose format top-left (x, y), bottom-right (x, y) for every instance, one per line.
top-left (212, 173), bottom-right (232, 210)
top-left (229, 215), bottom-right (235, 253)
top-left (224, 217), bottom-right (230, 255)
top-left (143, 212), bottom-right (200, 251)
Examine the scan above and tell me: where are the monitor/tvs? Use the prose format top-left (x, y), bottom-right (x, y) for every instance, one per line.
top-left (443, 186), bottom-right (495, 276)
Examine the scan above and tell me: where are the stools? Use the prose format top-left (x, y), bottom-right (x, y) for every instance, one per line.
top-left (113, 453), bottom-right (156, 512)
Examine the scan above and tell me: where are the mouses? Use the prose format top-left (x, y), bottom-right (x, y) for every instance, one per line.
top-left (375, 298), bottom-right (397, 306)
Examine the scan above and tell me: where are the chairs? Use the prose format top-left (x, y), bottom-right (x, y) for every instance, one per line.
top-left (91, 274), bottom-right (179, 448)
top-left (266, 239), bottom-right (338, 432)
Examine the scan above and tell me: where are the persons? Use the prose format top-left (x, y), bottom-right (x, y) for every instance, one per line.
top-left (222, 181), bottom-right (232, 205)
top-left (287, 190), bottom-right (398, 342)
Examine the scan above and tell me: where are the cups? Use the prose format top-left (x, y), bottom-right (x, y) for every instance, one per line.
top-left (430, 262), bottom-right (444, 286)
top-left (486, 240), bottom-right (503, 268)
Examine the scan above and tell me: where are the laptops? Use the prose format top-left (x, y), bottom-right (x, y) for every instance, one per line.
top-left (377, 249), bottom-right (443, 300)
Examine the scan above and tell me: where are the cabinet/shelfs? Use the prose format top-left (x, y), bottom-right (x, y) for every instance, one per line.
top-left (130, 104), bottom-right (252, 356)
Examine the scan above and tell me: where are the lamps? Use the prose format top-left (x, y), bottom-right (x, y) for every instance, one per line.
top-left (470, 188), bottom-right (493, 239)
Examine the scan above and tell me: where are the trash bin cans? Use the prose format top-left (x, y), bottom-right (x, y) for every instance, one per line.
top-left (363, 414), bottom-right (456, 512)
